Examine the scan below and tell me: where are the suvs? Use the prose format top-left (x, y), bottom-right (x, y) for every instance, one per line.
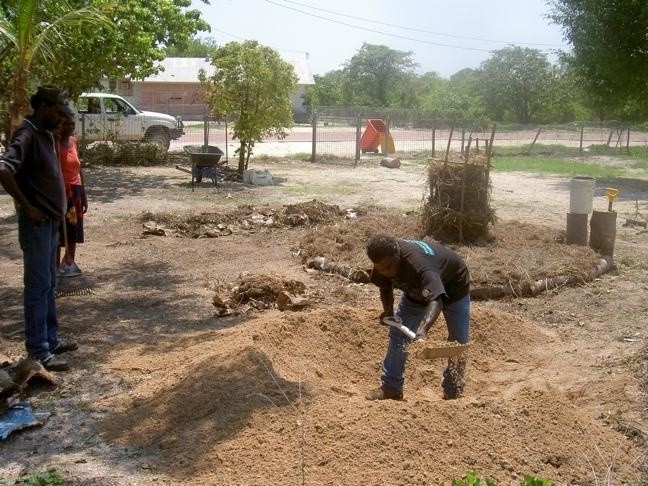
top-left (68, 91), bottom-right (187, 154)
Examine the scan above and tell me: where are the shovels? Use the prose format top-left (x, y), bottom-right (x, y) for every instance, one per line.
top-left (383, 319), bottom-right (471, 359)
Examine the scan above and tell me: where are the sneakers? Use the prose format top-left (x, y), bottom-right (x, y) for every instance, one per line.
top-left (364, 388), bottom-right (402, 399)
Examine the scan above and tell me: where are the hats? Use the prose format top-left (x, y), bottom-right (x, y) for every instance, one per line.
top-left (35, 83), bottom-right (76, 121)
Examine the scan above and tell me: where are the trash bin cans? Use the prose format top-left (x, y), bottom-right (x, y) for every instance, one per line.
top-left (569, 176), bottom-right (594, 214)
top-left (360, 119), bottom-right (386, 153)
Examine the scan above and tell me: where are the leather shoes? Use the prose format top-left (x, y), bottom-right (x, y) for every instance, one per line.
top-left (44, 356), bottom-right (69, 369)
top-left (54, 340), bottom-right (78, 353)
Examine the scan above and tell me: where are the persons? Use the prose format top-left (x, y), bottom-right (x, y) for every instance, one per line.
top-left (364, 235), bottom-right (469, 402)
top-left (0, 85), bottom-right (77, 370)
top-left (56, 105), bottom-right (88, 277)
